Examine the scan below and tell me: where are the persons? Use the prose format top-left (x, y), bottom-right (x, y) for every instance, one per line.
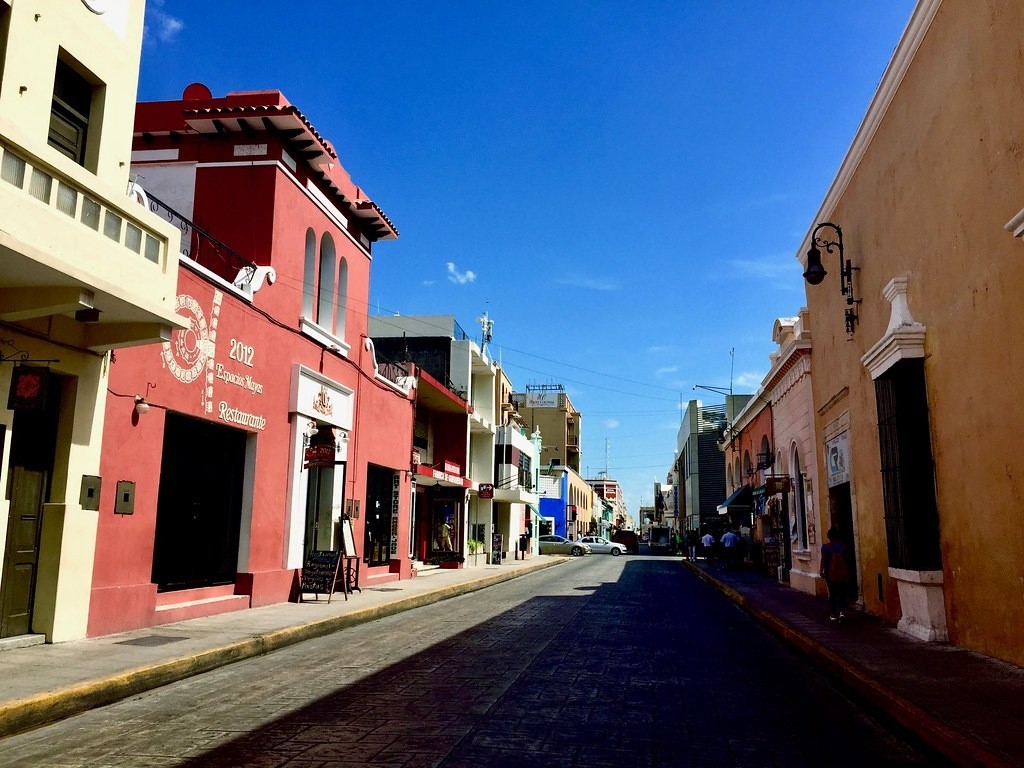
top-left (702, 530), bottom-right (715, 564)
top-left (440, 523), bottom-right (453, 551)
top-left (675, 530), bottom-right (683, 555)
top-left (720, 527), bottom-right (740, 569)
top-left (820, 525), bottom-right (849, 614)
top-left (684, 530), bottom-right (698, 563)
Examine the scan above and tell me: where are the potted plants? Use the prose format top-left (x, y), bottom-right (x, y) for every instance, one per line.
top-left (467, 537), bottom-right (486, 555)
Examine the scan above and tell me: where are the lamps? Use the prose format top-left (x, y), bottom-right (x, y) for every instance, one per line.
top-left (803, 221), bottom-right (863, 305)
top-left (717, 426), bottom-right (741, 452)
top-left (337, 431), bottom-right (350, 453)
top-left (300, 421), bottom-right (320, 474)
top-left (541, 445), bottom-right (559, 452)
top-left (133, 394), bottom-right (150, 414)
top-left (541, 447), bottom-right (548, 453)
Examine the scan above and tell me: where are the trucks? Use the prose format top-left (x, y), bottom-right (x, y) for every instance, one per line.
top-left (646, 525), bottom-right (672, 553)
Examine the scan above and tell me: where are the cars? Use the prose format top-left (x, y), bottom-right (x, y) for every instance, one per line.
top-left (538, 534), bottom-right (592, 556)
top-left (573, 537), bottom-right (629, 556)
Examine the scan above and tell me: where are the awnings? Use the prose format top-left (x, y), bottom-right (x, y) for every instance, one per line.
top-left (525, 503), bottom-right (547, 525)
top-left (719, 483), bottom-right (750, 508)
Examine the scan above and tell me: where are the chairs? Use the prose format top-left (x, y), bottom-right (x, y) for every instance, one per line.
top-left (552, 537), bottom-right (555, 542)
top-left (556, 537), bottom-right (560, 542)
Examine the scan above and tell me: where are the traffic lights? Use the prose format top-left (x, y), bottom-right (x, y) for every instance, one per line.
top-left (571, 506), bottom-right (577, 521)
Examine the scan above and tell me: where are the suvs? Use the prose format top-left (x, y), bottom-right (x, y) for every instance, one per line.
top-left (612, 530), bottom-right (640, 554)
